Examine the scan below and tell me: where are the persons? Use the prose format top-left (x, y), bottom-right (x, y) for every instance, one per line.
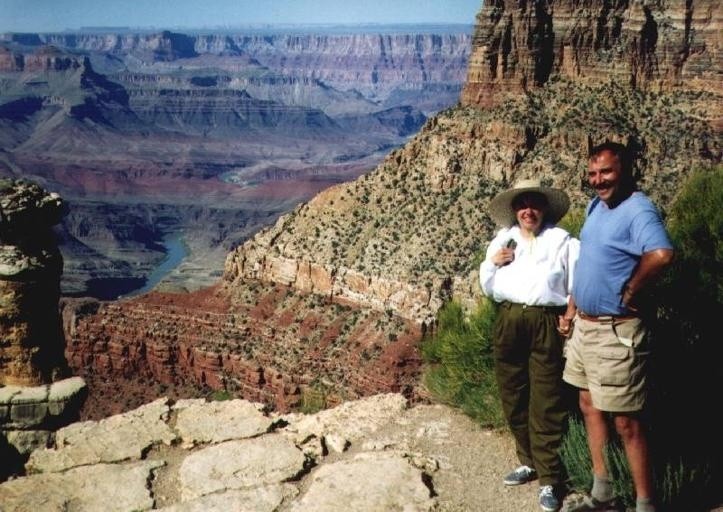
top-left (474, 177), bottom-right (582, 511)
top-left (559, 141), bottom-right (673, 511)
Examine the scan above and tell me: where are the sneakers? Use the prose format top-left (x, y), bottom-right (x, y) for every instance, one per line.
top-left (503, 465), bottom-right (538, 485)
top-left (538, 485), bottom-right (559, 511)
top-left (567, 492), bottom-right (622, 512)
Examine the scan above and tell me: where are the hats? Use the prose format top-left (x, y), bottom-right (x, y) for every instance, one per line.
top-left (487, 179), bottom-right (570, 227)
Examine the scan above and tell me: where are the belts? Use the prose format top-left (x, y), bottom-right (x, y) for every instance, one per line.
top-left (577, 311), bottom-right (634, 322)
